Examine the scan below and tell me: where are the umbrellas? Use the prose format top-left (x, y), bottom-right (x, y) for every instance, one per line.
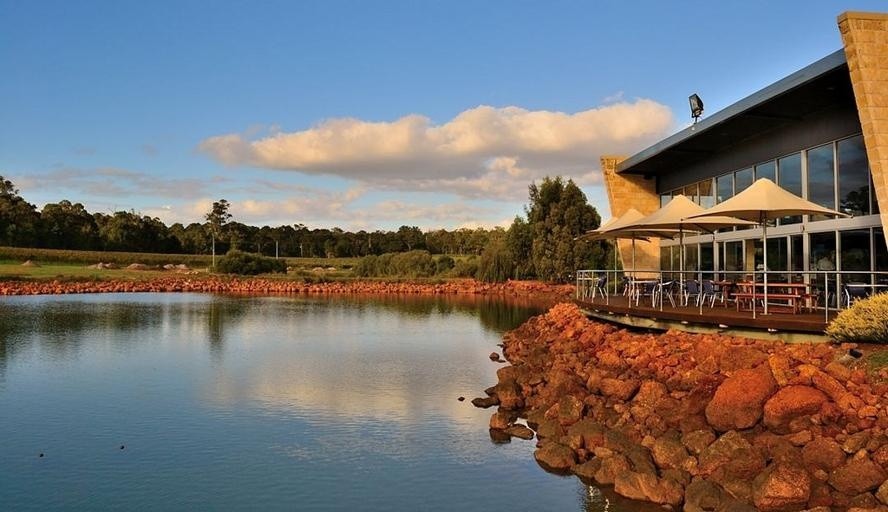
top-left (601, 193), bottom-right (776, 309)
top-left (679, 177), bottom-right (854, 315)
top-left (590, 208), bottom-right (674, 300)
top-left (574, 216), bottom-right (652, 294)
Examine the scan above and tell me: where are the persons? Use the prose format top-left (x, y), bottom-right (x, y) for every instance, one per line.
top-left (849, 258), bottom-right (869, 284)
top-left (815, 252), bottom-right (835, 287)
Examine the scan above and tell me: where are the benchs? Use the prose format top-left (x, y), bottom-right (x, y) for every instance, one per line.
top-left (731, 292), bottom-right (818, 300)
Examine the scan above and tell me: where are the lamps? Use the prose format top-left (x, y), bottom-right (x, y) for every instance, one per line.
top-left (690, 94), bottom-right (705, 124)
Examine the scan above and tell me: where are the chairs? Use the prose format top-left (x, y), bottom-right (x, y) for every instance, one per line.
top-left (581, 270), bottom-right (722, 309)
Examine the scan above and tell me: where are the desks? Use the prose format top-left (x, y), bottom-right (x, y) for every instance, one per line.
top-left (736, 282), bottom-right (811, 315)
